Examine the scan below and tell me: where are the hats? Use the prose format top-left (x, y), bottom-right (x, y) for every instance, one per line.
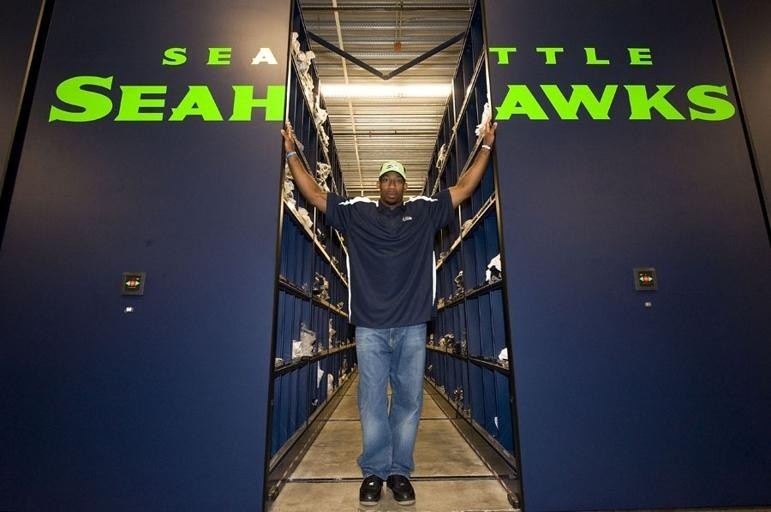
top-left (378, 160), bottom-right (408, 180)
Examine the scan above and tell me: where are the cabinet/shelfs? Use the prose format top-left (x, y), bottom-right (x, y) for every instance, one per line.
top-left (0, 0), bottom-right (358, 511)
top-left (418, 1), bottom-right (770, 512)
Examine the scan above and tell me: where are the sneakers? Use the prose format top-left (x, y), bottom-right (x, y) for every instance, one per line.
top-left (360, 474), bottom-right (383, 505)
top-left (386, 474), bottom-right (416, 505)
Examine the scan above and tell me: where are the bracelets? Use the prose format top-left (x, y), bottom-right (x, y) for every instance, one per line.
top-left (481, 144), bottom-right (492, 150)
top-left (286, 151), bottom-right (297, 159)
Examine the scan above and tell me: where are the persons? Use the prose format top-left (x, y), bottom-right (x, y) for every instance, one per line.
top-left (281, 115), bottom-right (498, 506)
